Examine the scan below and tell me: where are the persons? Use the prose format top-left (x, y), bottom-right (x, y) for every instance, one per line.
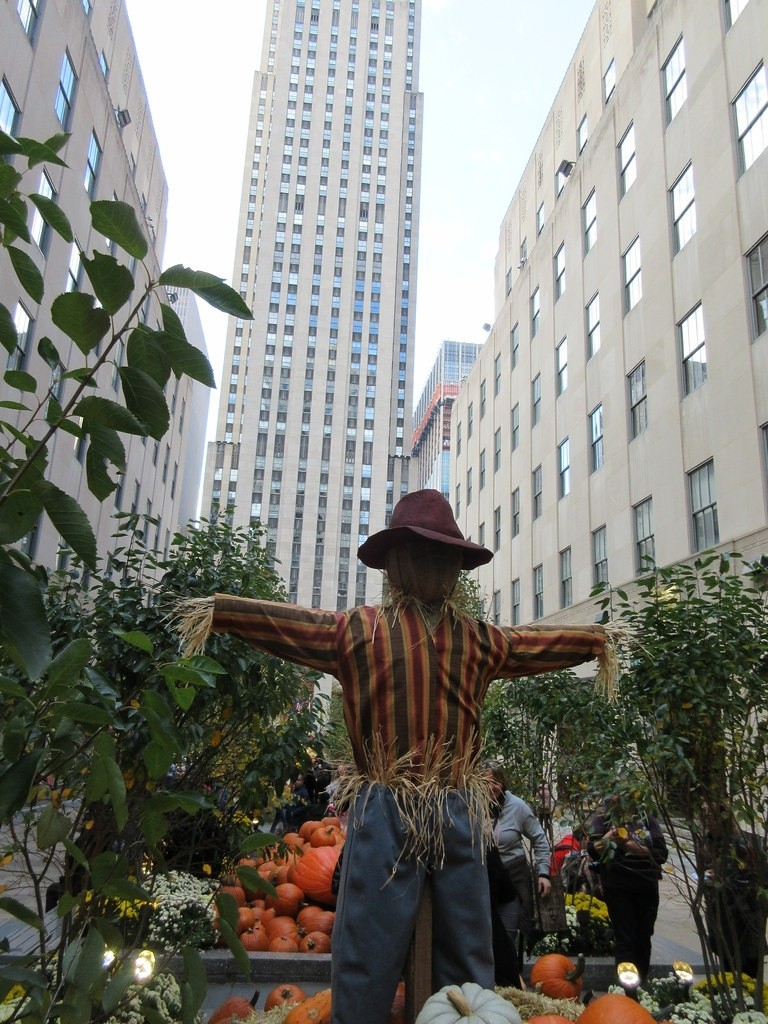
top-left (158, 490), bottom-right (625, 1024)
top-left (475, 759), bottom-right (768, 1004)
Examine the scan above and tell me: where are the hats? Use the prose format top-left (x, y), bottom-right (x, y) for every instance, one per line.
top-left (571, 824), bottom-right (591, 839)
top-left (357, 490), bottom-right (493, 571)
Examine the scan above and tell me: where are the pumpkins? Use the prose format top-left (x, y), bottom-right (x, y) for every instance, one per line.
top-left (206, 815), bottom-right (660, 1024)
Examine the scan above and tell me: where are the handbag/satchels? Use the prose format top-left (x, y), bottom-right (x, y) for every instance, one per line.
top-left (587, 839), bottom-right (625, 875)
top-left (530, 873), bottom-right (570, 934)
